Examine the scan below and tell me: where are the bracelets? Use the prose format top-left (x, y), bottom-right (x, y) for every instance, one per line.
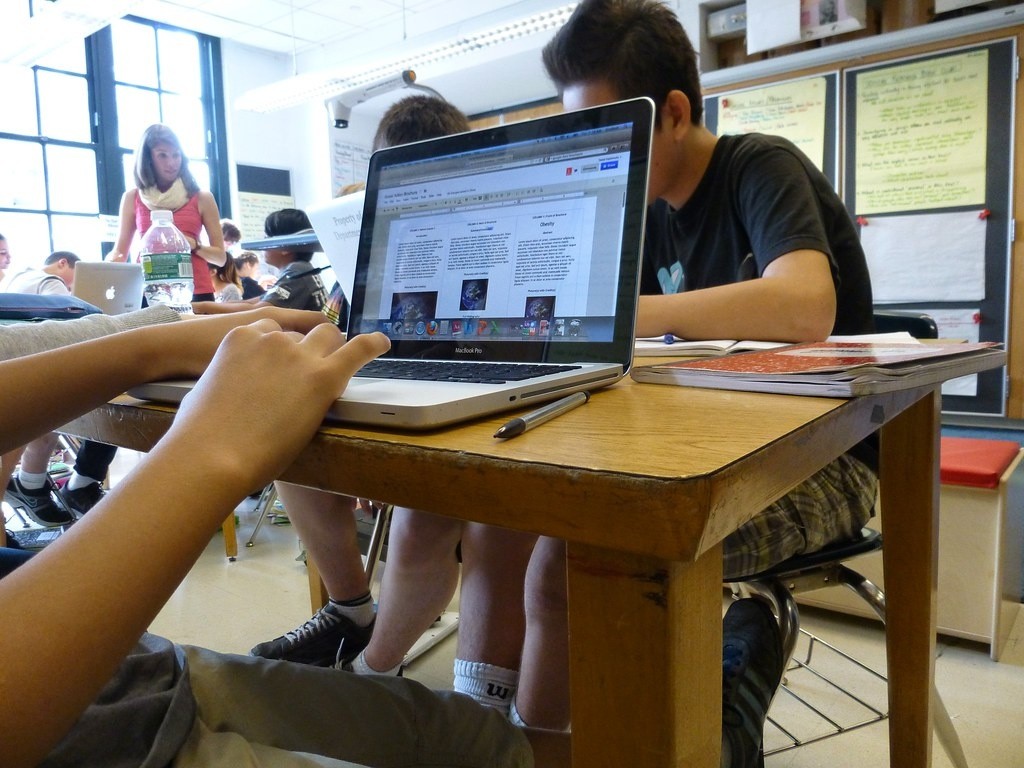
top-left (191, 238), bottom-right (202, 254)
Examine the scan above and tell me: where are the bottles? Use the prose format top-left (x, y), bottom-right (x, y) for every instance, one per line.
top-left (139, 210), bottom-right (194, 318)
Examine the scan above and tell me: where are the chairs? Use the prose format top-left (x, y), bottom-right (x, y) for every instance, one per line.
top-left (724, 309), bottom-right (969, 768)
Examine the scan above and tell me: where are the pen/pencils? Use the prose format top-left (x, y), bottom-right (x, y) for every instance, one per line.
top-left (492, 391), bottom-right (590, 439)
top-left (665, 333), bottom-right (673, 344)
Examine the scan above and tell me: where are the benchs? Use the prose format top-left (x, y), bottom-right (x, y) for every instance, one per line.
top-left (790, 434), bottom-right (1024, 661)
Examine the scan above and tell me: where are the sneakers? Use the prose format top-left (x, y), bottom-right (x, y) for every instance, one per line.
top-left (248, 603), bottom-right (379, 669)
top-left (719, 596), bottom-right (785, 768)
top-left (1, 474), bottom-right (72, 527)
top-left (339, 659), bottom-right (406, 678)
top-left (57, 479), bottom-right (107, 515)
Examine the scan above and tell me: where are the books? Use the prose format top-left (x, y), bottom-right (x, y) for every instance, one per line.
top-left (634, 331), bottom-right (921, 357)
top-left (630, 342), bottom-right (1007, 396)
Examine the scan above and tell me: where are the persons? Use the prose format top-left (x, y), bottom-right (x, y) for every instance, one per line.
top-left (0, 304), bottom-right (215, 578)
top-left (0, 251), bottom-right (81, 295)
top-left (452, 0), bottom-right (880, 734)
top-left (0, 232), bottom-right (10, 281)
top-left (190, 208), bottom-right (329, 314)
top-left (0, 304), bottom-right (784, 768)
top-left (104, 124), bottom-right (227, 308)
top-left (207, 218), bottom-right (278, 303)
top-left (248, 95), bottom-right (471, 676)
top-left (322, 181), bottom-right (366, 333)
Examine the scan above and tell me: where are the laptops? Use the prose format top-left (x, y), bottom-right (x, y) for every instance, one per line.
top-left (124, 96), bottom-right (657, 432)
top-left (73, 261), bottom-right (143, 316)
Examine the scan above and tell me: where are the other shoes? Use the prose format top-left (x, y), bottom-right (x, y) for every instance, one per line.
top-left (4, 528), bottom-right (25, 549)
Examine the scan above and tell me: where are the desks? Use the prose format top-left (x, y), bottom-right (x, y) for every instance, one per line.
top-left (55, 371), bottom-right (942, 768)
